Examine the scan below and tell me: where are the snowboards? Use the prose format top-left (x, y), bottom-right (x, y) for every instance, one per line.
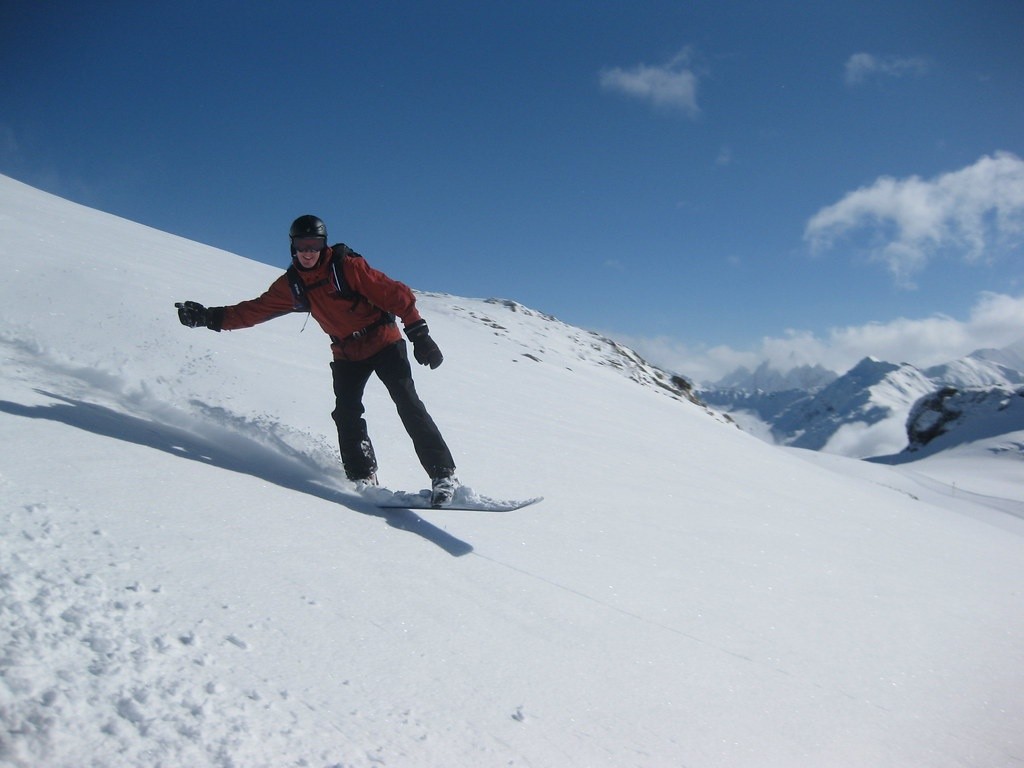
top-left (370, 491), bottom-right (549, 514)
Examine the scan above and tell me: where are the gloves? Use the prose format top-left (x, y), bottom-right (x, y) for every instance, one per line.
top-left (403, 319), bottom-right (443, 369)
top-left (175, 301), bottom-right (223, 332)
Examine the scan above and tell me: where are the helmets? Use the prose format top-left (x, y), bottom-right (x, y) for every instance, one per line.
top-left (289, 215), bottom-right (327, 257)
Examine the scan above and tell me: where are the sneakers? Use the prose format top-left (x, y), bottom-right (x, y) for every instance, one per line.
top-left (351, 473), bottom-right (379, 492)
top-left (431, 475), bottom-right (460, 505)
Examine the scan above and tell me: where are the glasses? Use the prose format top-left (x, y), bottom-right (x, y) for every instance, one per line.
top-left (292, 237), bottom-right (325, 252)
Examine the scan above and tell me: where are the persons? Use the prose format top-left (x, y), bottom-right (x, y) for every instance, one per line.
top-left (173, 214), bottom-right (462, 508)
